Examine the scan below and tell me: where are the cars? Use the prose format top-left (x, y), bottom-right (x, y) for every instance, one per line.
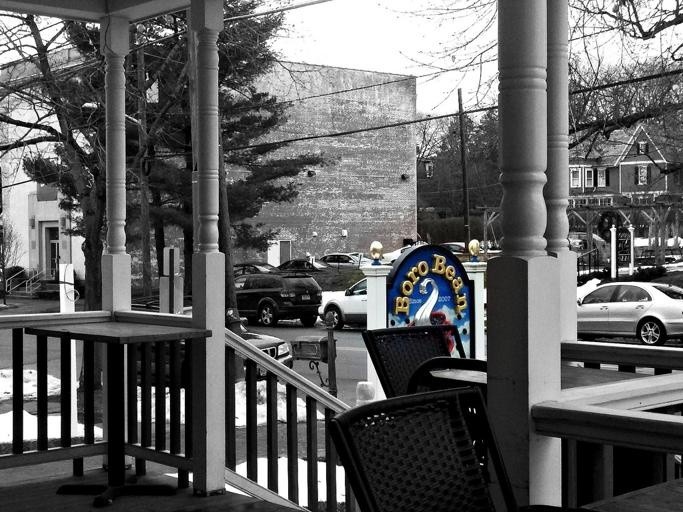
top-left (232, 241), bottom-right (466, 281)
top-left (568, 231), bottom-right (605, 250)
top-left (576, 281), bottom-right (682, 345)
top-left (317, 277), bottom-right (367, 331)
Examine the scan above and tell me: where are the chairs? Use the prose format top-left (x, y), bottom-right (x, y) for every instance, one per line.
top-left (330, 387), bottom-right (569, 510)
top-left (360, 326), bottom-right (488, 410)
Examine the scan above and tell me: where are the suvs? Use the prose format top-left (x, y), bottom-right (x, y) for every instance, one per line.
top-left (234, 270), bottom-right (323, 329)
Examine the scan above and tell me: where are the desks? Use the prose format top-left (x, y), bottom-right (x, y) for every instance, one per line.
top-left (429, 363), bottom-right (655, 388)
top-left (581, 476), bottom-right (683, 512)
top-left (19, 323), bottom-right (211, 509)
